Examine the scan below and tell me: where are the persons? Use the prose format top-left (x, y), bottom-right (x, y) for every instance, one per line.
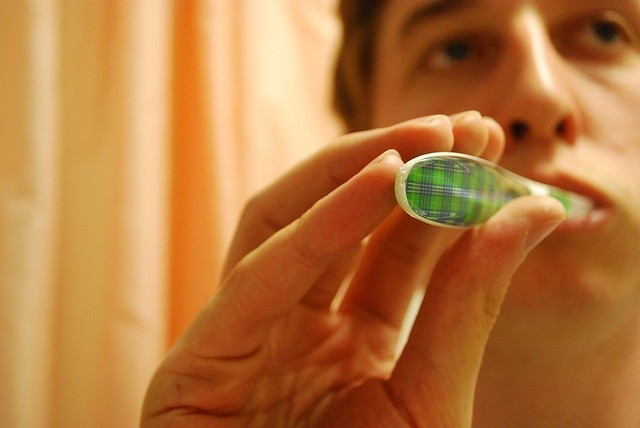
top-left (141, 0), bottom-right (640, 428)
